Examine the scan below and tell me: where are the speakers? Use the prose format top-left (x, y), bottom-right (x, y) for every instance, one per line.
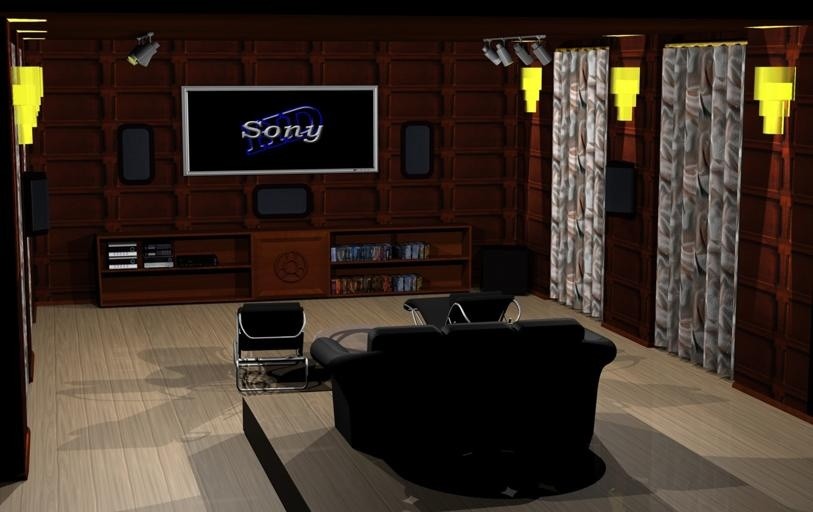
top-left (117, 123), bottom-right (155, 185)
top-left (21, 171), bottom-right (49, 236)
top-left (400, 120), bottom-right (435, 179)
top-left (605, 159), bottom-right (636, 219)
top-left (251, 183), bottom-right (314, 219)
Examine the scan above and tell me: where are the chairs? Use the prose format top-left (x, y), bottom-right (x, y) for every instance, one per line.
top-left (232, 300), bottom-right (310, 394)
top-left (401, 289), bottom-right (520, 327)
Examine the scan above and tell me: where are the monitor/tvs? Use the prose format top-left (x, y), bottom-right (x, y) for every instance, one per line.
top-left (181, 85), bottom-right (379, 178)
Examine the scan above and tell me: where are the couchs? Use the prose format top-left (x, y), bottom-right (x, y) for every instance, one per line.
top-left (312, 317), bottom-right (618, 482)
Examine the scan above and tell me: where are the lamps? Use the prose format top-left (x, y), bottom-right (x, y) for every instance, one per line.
top-left (481, 34), bottom-right (553, 114)
top-left (610, 65), bottom-right (643, 124)
top-left (127, 32), bottom-right (159, 67)
top-left (14, 61), bottom-right (45, 149)
top-left (752, 64), bottom-right (800, 137)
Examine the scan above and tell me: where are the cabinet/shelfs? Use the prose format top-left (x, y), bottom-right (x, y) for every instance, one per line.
top-left (96, 220), bottom-right (475, 306)
